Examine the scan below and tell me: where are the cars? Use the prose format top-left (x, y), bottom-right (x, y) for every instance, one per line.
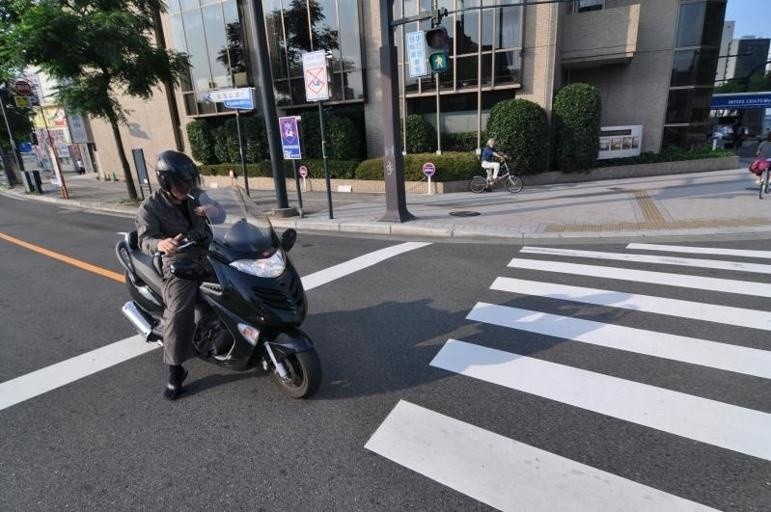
top-left (687, 124), bottom-right (752, 153)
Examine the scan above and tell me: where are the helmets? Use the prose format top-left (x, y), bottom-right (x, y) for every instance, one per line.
top-left (155, 151), bottom-right (201, 202)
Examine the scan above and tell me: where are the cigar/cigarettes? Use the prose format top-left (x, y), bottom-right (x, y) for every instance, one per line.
top-left (187, 193), bottom-right (195, 200)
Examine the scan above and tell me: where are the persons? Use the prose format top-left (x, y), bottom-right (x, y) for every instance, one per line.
top-left (135, 151), bottom-right (226, 401)
top-left (756, 132), bottom-right (771, 190)
top-left (77, 159), bottom-right (84, 175)
top-left (481, 138), bottom-right (503, 192)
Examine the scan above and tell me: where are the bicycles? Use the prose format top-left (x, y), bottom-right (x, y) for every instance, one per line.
top-left (471, 154), bottom-right (524, 193)
top-left (755, 166), bottom-right (770, 199)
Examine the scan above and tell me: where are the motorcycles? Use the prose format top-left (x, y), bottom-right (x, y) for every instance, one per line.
top-left (111, 181), bottom-right (325, 399)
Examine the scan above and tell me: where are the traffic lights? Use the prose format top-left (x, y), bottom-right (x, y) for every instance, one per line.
top-left (426, 27), bottom-right (451, 53)
top-left (429, 53), bottom-right (451, 74)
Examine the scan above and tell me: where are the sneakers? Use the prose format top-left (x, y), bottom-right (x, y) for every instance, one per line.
top-left (162, 365), bottom-right (189, 402)
